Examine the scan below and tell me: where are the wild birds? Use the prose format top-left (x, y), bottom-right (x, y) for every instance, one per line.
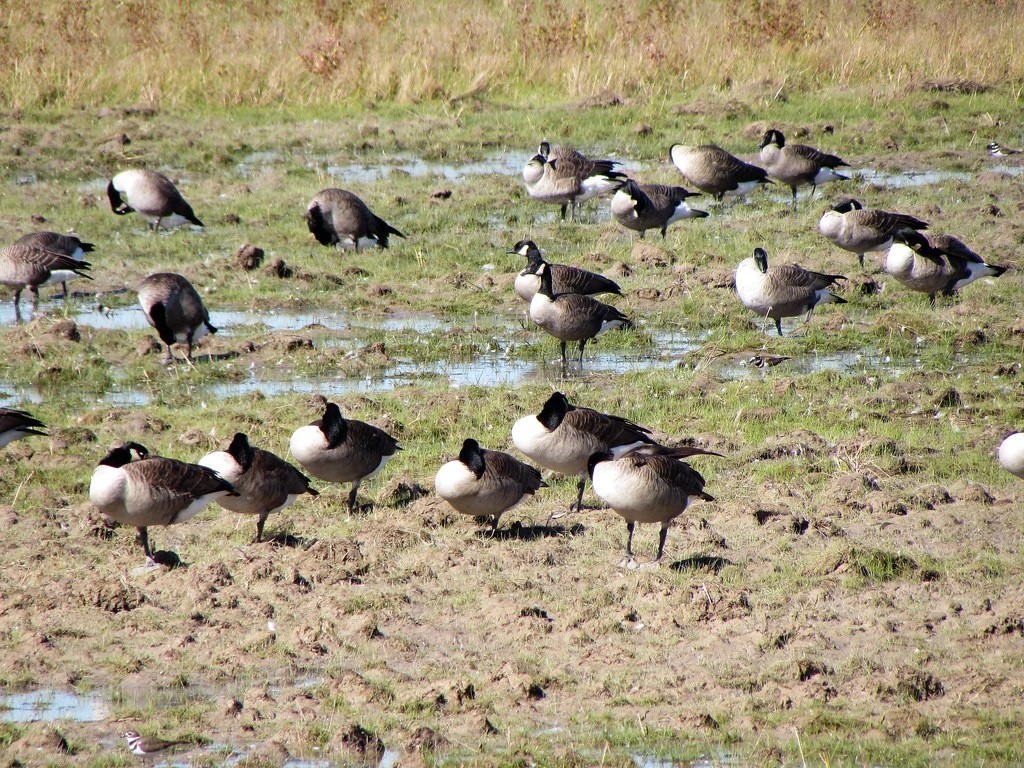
top-left (106, 168), bottom-right (209, 235)
top-left (88, 441), bottom-right (243, 567)
top-left (511, 390), bottom-right (728, 519)
top-left (505, 239), bottom-right (625, 361)
top-left (0, 405), bottom-right (49, 452)
top-left (306, 187), bottom-right (408, 257)
top-left (748, 355), bottom-right (791, 379)
top-left (196, 433), bottom-right (319, 548)
top-left (886, 227), bottom-right (1010, 311)
top-left (290, 402), bottom-right (407, 521)
top-left (586, 450), bottom-right (716, 572)
top-left (435, 438), bottom-right (551, 537)
top-left (735, 246), bottom-right (849, 335)
top-left (613, 178), bottom-right (709, 242)
top-left (758, 129), bottom-right (852, 213)
top-left (819, 198), bottom-right (930, 275)
top-left (522, 140), bottom-right (630, 224)
top-left (520, 258), bottom-right (631, 366)
top-left (986, 142), bottom-right (1022, 167)
top-left (0, 231), bottom-right (97, 309)
top-left (139, 272), bottom-right (218, 371)
top-left (668, 143), bottom-right (778, 212)
